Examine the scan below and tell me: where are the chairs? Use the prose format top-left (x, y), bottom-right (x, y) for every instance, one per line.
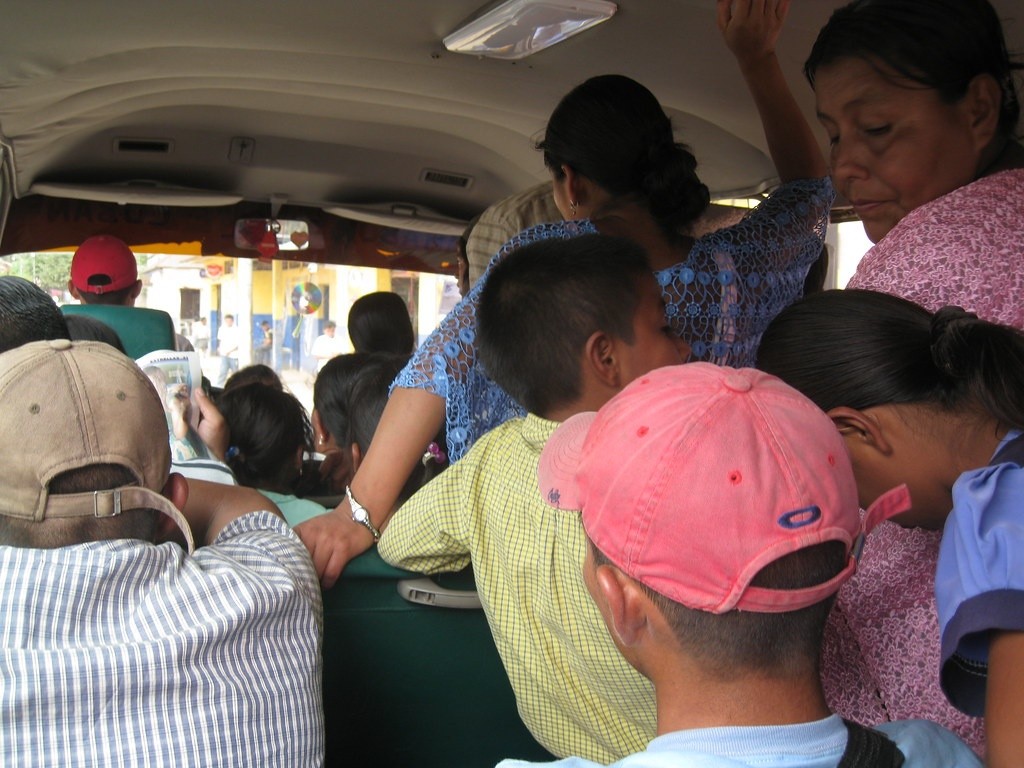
top-left (320, 548), bottom-right (561, 768)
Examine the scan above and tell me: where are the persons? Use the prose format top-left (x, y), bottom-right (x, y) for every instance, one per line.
top-left (0, 0), bottom-right (1024, 767)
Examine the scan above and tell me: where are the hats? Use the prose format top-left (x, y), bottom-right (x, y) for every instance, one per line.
top-left (0, 339), bottom-right (195, 556)
top-left (71, 234), bottom-right (138, 294)
top-left (537, 362), bottom-right (913, 614)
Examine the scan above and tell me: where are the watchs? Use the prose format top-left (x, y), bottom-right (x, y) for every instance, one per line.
top-left (343, 485), bottom-right (382, 541)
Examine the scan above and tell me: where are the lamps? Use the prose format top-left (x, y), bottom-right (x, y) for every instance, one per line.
top-left (442, 0), bottom-right (618, 61)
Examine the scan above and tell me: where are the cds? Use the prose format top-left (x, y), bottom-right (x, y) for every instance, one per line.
top-left (292, 283), bottom-right (323, 314)
